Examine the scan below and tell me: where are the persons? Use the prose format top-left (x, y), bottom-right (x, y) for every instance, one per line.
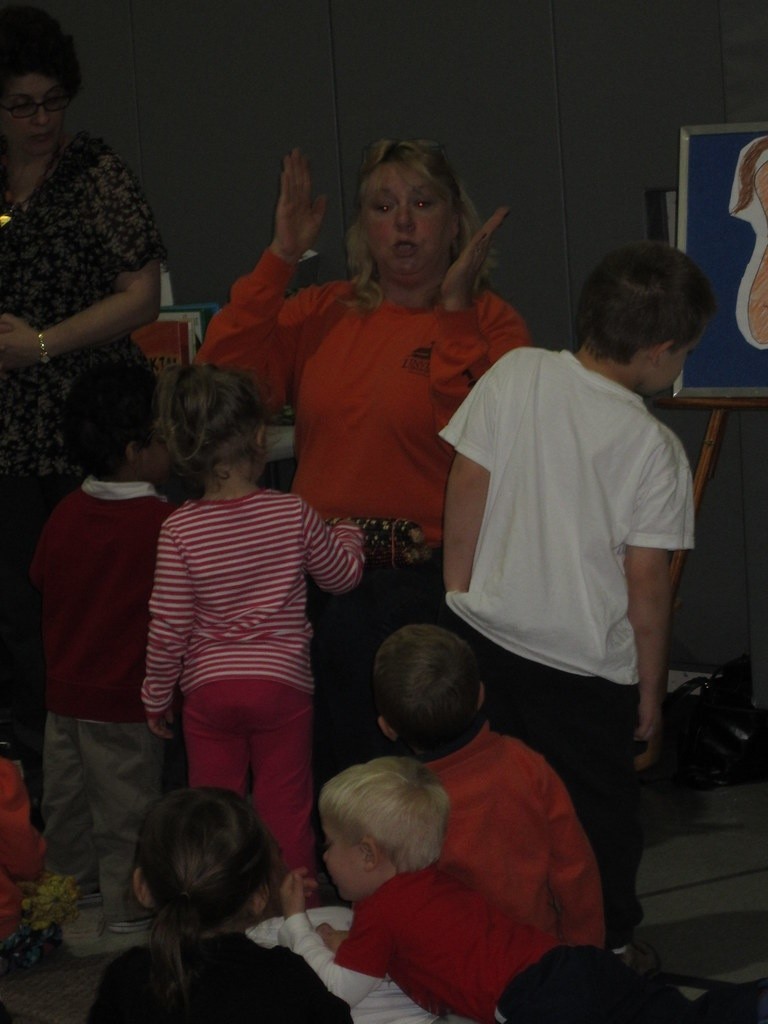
top-left (0, 3), bottom-right (167, 832)
top-left (432, 238), bottom-right (716, 977)
top-left (194, 134), bottom-right (535, 895)
top-left (280, 755), bottom-right (768, 1024)
top-left (31, 361), bottom-right (180, 932)
top-left (374, 625), bottom-right (610, 949)
top-left (138, 362), bottom-right (366, 907)
top-left (0, 754), bottom-right (65, 976)
top-left (86, 787), bottom-right (352, 1024)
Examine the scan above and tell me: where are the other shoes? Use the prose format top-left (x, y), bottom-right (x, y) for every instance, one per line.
top-left (108, 917), bottom-right (154, 933)
top-left (75, 893), bottom-right (102, 904)
top-left (618, 939), bottom-right (656, 975)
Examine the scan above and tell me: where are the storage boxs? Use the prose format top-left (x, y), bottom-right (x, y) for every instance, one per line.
top-left (130, 301), bottom-right (219, 368)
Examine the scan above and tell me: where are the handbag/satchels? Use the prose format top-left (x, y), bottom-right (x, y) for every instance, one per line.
top-left (663, 653), bottom-right (768, 789)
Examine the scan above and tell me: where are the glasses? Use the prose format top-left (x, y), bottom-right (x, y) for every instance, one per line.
top-left (360, 137), bottom-right (449, 162)
top-left (0, 93), bottom-right (72, 119)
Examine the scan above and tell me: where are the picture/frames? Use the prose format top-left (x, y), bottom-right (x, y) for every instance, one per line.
top-left (667, 120), bottom-right (768, 398)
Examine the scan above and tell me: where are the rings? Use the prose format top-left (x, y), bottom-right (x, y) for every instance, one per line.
top-left (473, 248), bottom-right (482, 252)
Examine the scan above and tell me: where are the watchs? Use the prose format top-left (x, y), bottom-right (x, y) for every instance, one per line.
top-left (38, 333), bottom-right (51, 364)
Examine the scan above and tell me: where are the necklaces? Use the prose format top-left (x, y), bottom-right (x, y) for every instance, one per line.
top-left (0, 160), bottom-right (50, 227)
